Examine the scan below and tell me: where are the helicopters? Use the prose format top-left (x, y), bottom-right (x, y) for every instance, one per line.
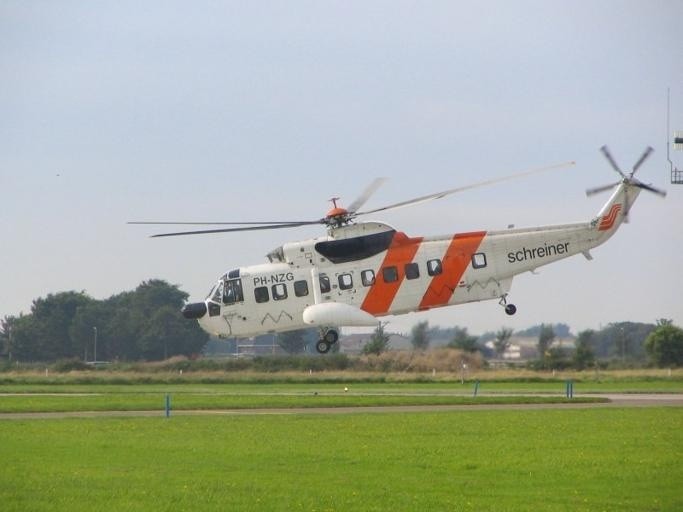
top-left (126, 142), bottom-right (666, 354)
